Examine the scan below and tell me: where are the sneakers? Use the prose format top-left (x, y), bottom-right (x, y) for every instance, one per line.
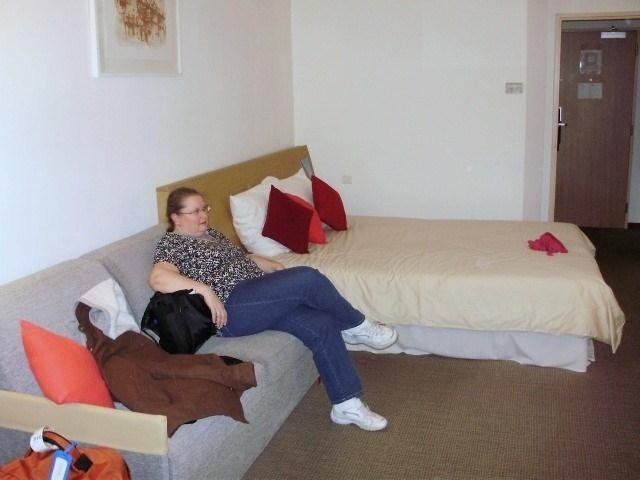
top-left (330, 398), bottom-right (388, 432)
top-left (341, 318), bottom-right (398, 350)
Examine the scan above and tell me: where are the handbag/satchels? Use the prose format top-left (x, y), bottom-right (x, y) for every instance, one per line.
top-left (140, 291), bottom-right (218, 355)
top-left (0, 424), bottom-right (131, 480)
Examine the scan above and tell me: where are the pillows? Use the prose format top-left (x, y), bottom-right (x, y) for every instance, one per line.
top-left (230, 176), bottom-right (290, 256)
top-left (280, 167), bottom-right (313, 202)
top-left (262, 185), bottom-right (313, 254)
top-left (285, 193), bottom-right (329, 244)
top-left (311, 175), bottom-right (347, 231)
top-left (20, 319), bottom-right (116, 409)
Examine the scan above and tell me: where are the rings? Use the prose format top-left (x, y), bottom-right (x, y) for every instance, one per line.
top-left (216, 310), bottom-right (221, 315)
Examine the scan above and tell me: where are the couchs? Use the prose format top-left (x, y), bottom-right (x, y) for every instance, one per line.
top-left (0, 222), bottom-right (320, 480)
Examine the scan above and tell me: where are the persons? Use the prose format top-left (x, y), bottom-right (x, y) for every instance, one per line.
top-left (148, 187), bottom-right (399, 433)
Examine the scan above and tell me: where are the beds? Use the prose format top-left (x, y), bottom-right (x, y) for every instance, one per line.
top-left (157, 145), bottom-right (626, 372)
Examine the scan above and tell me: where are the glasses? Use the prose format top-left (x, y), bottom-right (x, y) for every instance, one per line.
top-left (176, 204), bottom-right (212, 215)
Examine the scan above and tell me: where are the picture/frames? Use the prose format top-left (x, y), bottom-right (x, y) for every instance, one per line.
top-left (88, 0), bottom-right (182, 77)
